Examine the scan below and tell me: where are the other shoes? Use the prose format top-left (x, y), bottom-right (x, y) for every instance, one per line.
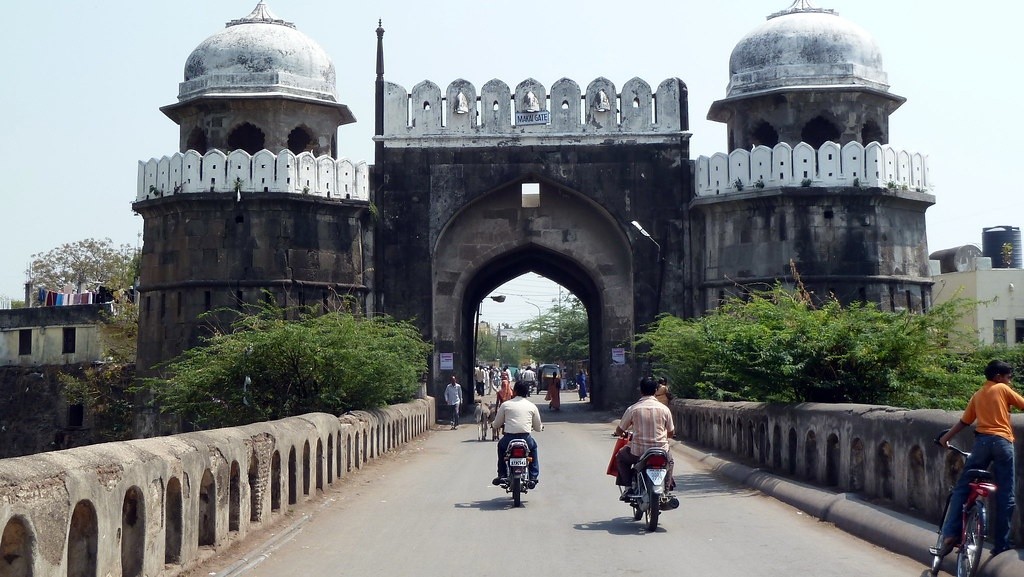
top-left (989, 548), bottom-right (1004, 557)
top-left (492, 475), bottom-right (507, 486)
top-left (619, 487), bottom-right (633, 500)
top-left (450, 425), bottom-right (459, 430)
top-left (549, 405), bottom-right (552, 409)
top-left (938, 536), bottom-right (958, 556)
top-left (528, 480), bottom-right (536, 489)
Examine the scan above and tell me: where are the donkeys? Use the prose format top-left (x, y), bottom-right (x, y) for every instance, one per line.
top-left (474, 402), bottom-right (500, 441)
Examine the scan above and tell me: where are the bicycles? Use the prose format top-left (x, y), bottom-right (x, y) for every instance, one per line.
top-left (930, 429), bottom-right (998, 577)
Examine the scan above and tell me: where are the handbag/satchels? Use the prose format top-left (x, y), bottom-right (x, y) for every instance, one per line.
top-left (607, 432), bottom-right (632, 477)
top-left (545, 391), bottom-right (551, 401)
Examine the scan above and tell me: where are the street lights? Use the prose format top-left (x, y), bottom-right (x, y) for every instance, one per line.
top-left (523, 300), bottom-right (541, 356)
top-left (474, 295), bottom-right (506, 373)
top-left (631, 221), bottom-right (666, 377)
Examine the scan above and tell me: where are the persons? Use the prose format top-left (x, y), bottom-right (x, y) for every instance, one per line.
top-left (548, 371), bottom-right (561, 411)
top-left (444, 376), bottom-right (463, 430)
top-left (576, 369), bottom-right (587, 401)
top-left (495, 380), bottom-right (513, 439)
top-left (614, 376), bottom-right (675, 500)
top-left (492, 380), bottom-right (543, 486)
top-left (654, 378), bottom-right (673, 407)
top-left (939, 360), bottom-right (1024, 556)
top-left (474, 363), bottom-right (536, 397)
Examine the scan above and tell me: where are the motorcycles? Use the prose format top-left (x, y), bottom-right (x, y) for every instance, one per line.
top-left (525, 382), bottom-right (535, 397)
top-left (611, 433), bottom-right (680, 532)
top-left (492, 421), bottom-right (545, 507)
top-left (536, 363), bottom-right (561, 395)
